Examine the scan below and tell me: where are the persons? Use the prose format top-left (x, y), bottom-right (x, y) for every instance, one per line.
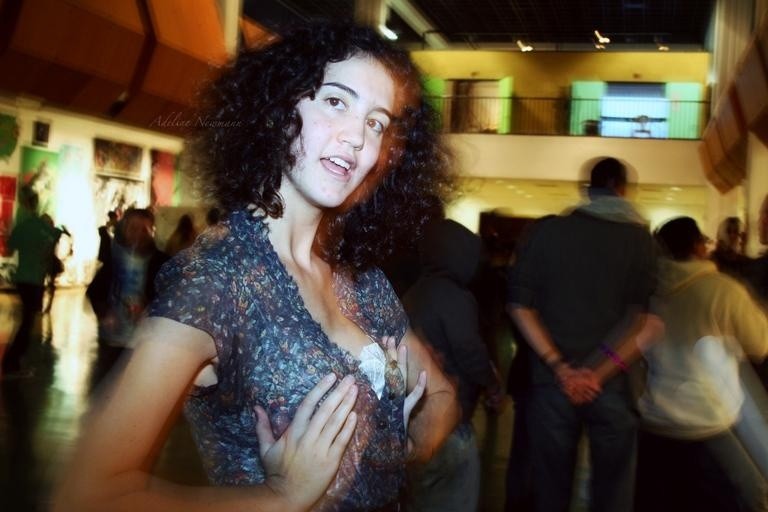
top-left (1, 174), bottom-right (62, 334)
top-left (381, 221), bottom-right (516, 510)
top-left (86, 209), bottom-right (196, 388)
top-left (2, 18), bottom-right (767, 511)
top-left (507, 156), bottom-right (768, 511)
top-left (71, 18), bottom-right (458, 512)
